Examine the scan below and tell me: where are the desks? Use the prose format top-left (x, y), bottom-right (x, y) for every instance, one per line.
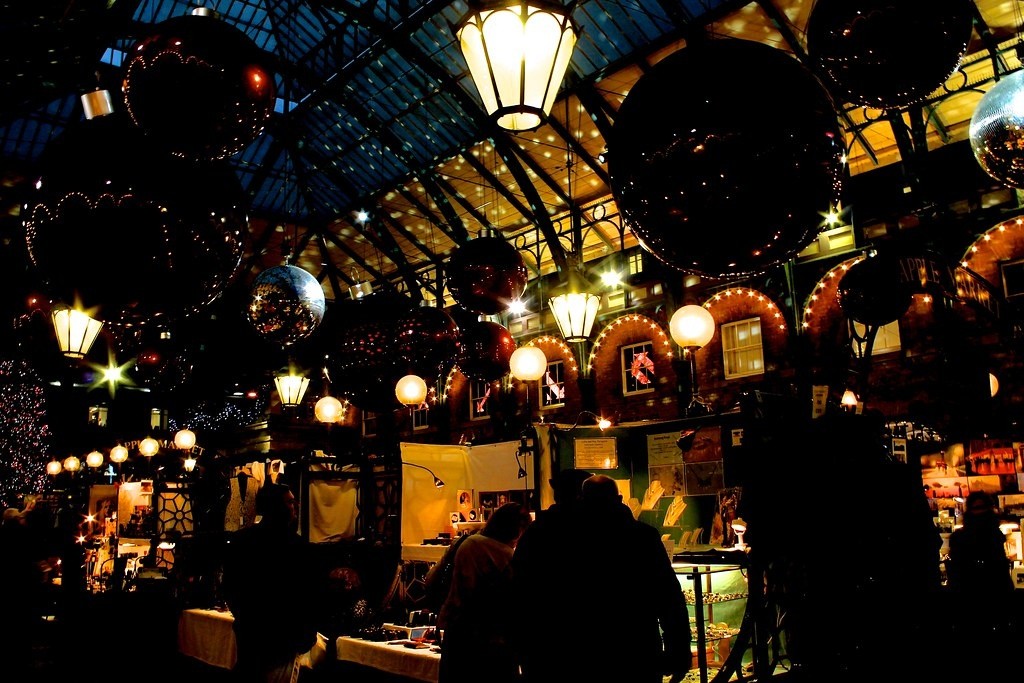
top-left (335, 636), bottom-right (442, 683)
top-left (177, 608), bottom-right (328, 670)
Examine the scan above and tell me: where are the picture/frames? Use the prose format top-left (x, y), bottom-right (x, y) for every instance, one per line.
top-left (449, 489), bottom-right (531, 523)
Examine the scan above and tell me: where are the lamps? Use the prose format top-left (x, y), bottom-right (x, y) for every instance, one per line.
top-left (0, 0), bottom-right (1024, 487)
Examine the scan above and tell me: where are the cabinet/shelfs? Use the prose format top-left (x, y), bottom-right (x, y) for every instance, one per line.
top-left (663, 565), bottom-right (786, 683)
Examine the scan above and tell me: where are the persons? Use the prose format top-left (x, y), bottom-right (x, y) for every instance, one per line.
top-left (0, 498), bottom-right (114, 577)
top-left (435, 502), bottom-right (532, 683)
top-left (946, 492), bottom-right (1015, 586)
top-left (515, 469), bottom-right (692, 683)
top-left (220, 484), bottom-right (366, 683)
